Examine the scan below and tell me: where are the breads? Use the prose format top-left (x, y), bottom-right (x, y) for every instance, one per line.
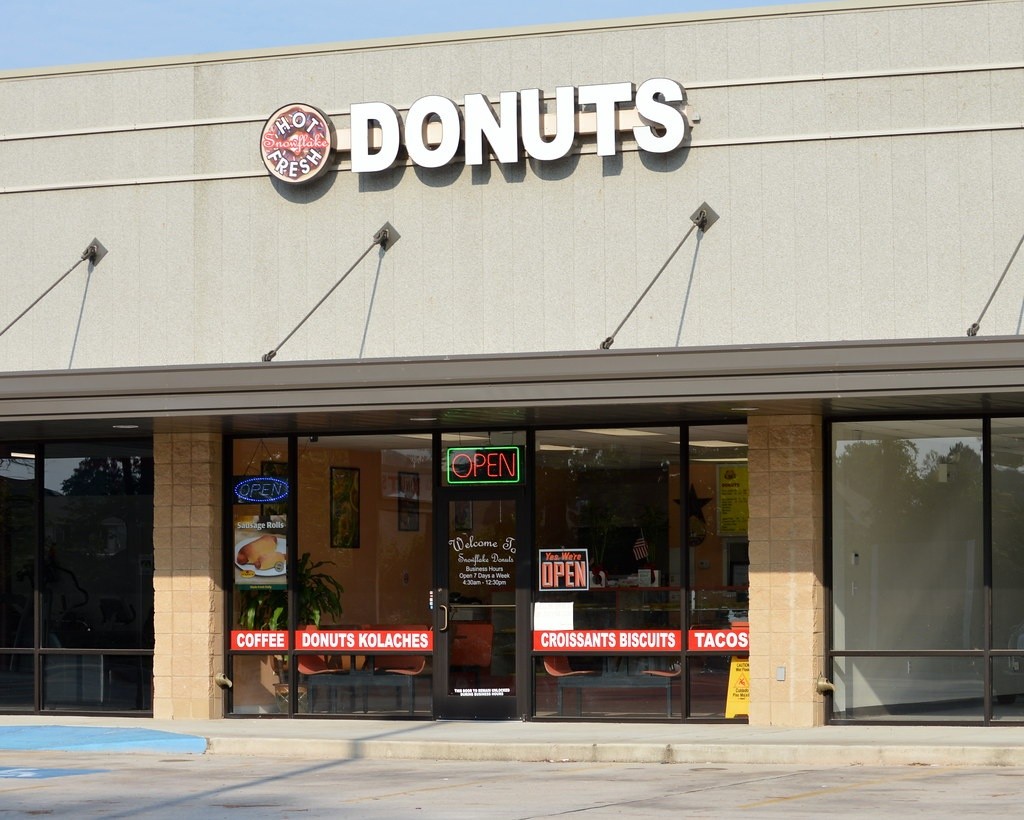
top-left (237, 535), bottom-right (286, 569)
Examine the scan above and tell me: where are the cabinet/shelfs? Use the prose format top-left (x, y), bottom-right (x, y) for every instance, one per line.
top-left (539, 585), bottom-right (746, 611)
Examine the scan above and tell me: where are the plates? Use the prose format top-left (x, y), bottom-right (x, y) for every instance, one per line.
top-left (235, 537), bottom-right (286, 577)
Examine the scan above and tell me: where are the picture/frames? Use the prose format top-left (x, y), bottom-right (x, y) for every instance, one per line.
top-left (329, 466), bottom-right (361, 550)
top-left (397, 471), bottom-right (420, 532)
top-left (259, 460), bottom-right (286, 535)
top-left (452, 502), bottom-right (473, 533)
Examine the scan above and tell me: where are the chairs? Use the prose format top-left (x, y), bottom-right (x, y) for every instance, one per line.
top-left (689, 624), bottom-right (714, 674)
top-left (544, 656), bottom-right (597, 718)
top-left (447, 622), bottom-right (495, 692)
top-left (640, 657), bottom-right (682, 717)
top-left (296, 625), bottom-right (348, 713)
top-left (361, 623), bottom-right (427, 712)
